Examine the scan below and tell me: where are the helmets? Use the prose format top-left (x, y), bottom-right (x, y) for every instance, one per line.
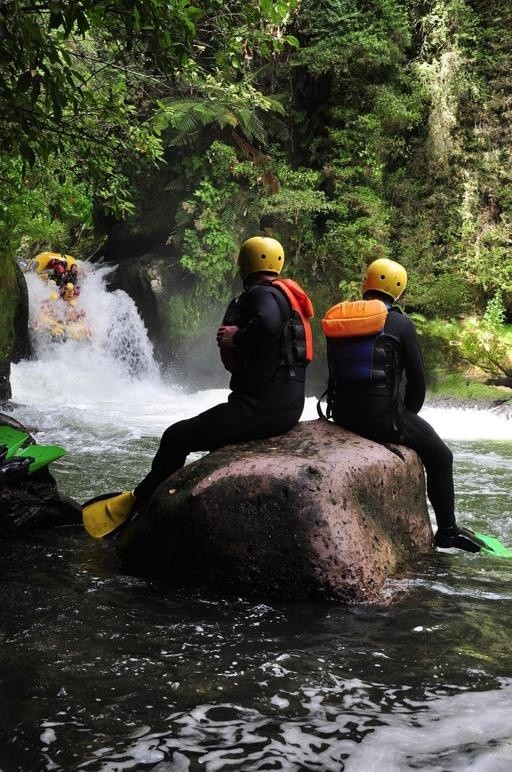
top-left (237, 235), bottom-right (285, 282)
top-left (361, 257), bottom-right (409, 302)
top-left (49, 255), bottom-right (80, 307)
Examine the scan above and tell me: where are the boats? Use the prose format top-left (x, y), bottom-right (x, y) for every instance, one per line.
top-left (23, 251), bottom-right (90, 338)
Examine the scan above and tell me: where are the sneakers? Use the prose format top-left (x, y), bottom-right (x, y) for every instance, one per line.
top-left (433, 523), bottom-right (479, 553)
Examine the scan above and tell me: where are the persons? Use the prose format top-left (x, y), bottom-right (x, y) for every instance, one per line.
top-left (323, 255), bottom-right (510, 557)
top-left (77, 234), bottom-right (316, 538)
top-left (45, 260), bottom-right (87, 321)
